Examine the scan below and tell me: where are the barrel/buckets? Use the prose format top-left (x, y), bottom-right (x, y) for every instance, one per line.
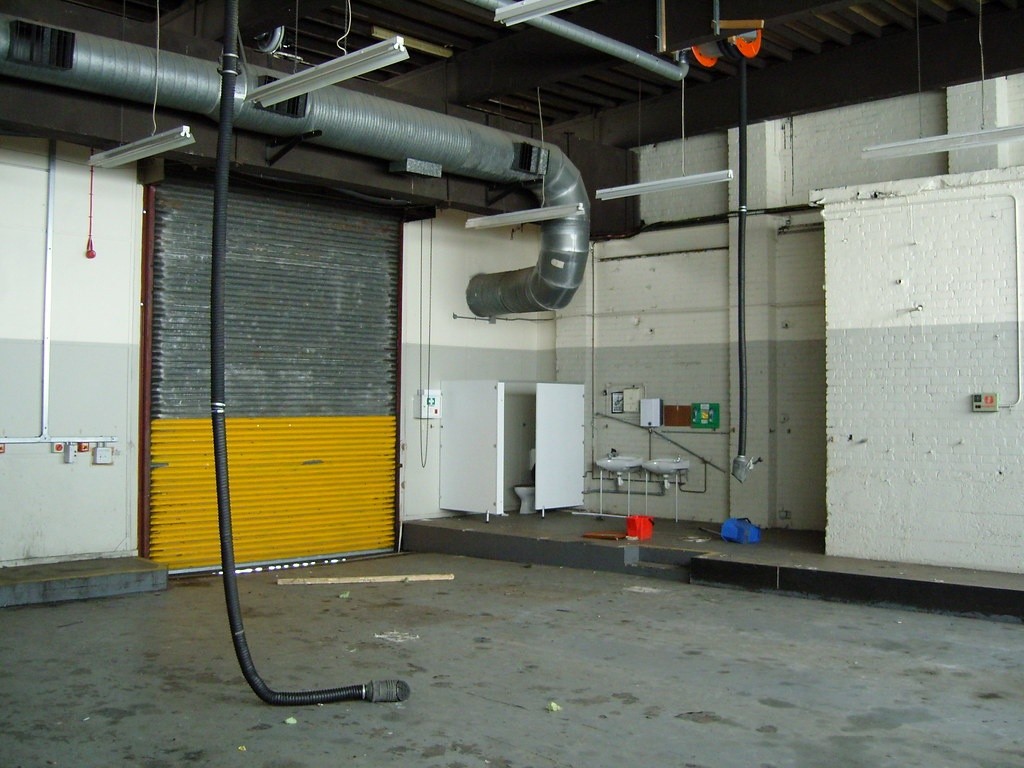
top-left (626, 514), bottom-right (655, 541)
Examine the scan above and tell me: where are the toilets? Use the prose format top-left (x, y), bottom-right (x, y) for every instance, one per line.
top-left (513, 482), bottom-right (541, 515)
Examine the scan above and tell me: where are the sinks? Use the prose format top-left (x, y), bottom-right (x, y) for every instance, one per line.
top-left (595, 455), bottom-right (645, 471)
top-left (643, 457), bottom-right (690, 476)
